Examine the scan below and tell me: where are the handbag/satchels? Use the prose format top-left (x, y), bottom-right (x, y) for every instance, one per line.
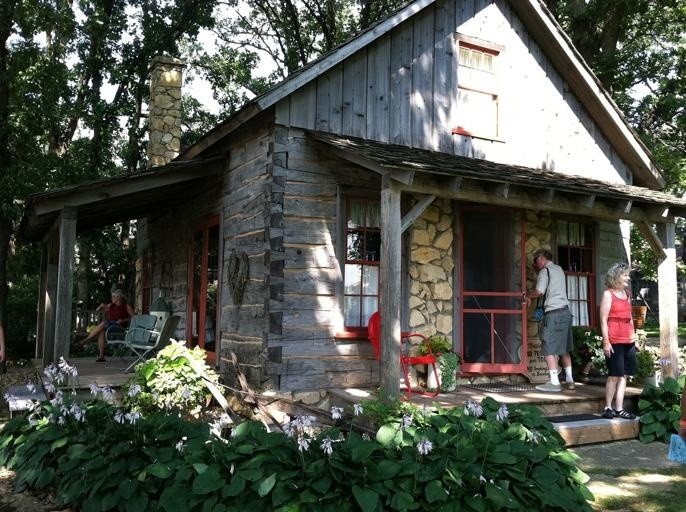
top-left (534, 307), bottom-right (544, 320)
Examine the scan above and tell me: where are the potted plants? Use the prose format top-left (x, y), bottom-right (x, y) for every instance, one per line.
top-left (635, 349), bottom-right (657, 386)
top-left (419, 332), bottom-right (461, 393)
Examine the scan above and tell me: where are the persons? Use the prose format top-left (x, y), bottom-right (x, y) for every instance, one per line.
top-left (527, 249), bottom-right (577, 391)
top-left (597, 262), bottom-right (641, 419)
top-left (76, 284), bottom-right (134, 363)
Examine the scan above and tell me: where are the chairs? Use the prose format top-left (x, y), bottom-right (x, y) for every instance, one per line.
top-left (105, 314), bottom-right (181, 374)
top-left (368, 310), bottom-right (441, 401)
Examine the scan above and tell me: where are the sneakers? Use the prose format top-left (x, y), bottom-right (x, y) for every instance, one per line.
top-left (95, 354), bottom-right (105, 362)
top-left (561, 381), bottom-right (575, 389)
top-left (73, 342), bottom-right (83, 349)
top-left (612, 408), bottom-right (636, 419)
top-left (535, 381), bottom-right (562, 392)
top-left (602, 408), bottom-right (613, 418)
